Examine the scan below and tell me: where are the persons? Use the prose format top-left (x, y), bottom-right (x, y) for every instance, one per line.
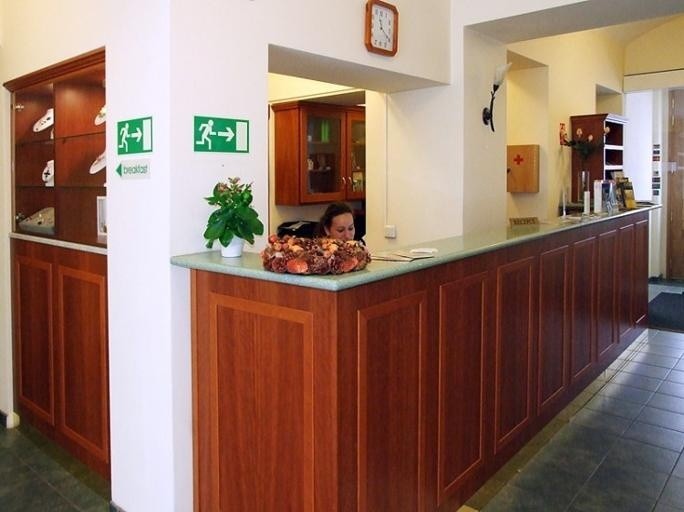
top-left (314, 202), bottom-right (355, 240)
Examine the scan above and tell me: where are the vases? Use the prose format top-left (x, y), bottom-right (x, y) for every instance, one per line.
top-left (578, 170), bottom-right (591, 202)
top-left (220, 235), bottom-right (245, 257)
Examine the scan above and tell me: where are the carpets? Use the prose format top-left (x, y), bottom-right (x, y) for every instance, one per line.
top-left (644, 292), bottom-right (684, 335)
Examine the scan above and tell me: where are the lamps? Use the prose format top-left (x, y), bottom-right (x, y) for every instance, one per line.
top-left (482, 62), bottom-right (512, 132)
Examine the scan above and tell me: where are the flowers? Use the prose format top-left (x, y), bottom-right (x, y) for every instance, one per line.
top-left (203, 177), bottom-right (264, 249)
top-left (559, 121), bottom-right (610, 173)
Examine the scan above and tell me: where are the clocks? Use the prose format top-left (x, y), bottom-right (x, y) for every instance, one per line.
top-left (365, 0), bottom-right (399, 56)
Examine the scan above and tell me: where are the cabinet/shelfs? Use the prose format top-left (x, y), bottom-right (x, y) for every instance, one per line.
top-left (569, 113), bottom-right (629, 204)
top-left (271, 101), bottom-right (366, 205)
top-left (2, 47), bottom-right (110, 485)
